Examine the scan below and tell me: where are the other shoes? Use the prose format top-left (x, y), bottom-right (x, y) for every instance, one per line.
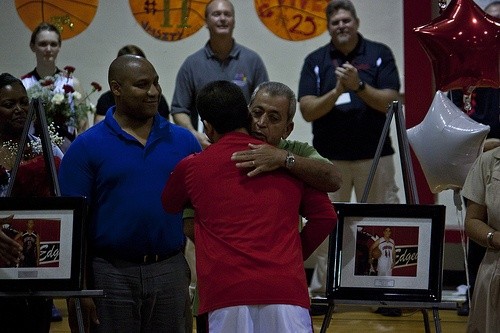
top-left (373, 306), bottom-right (401, 318)
top-left (51, 305), bottom-right (62, 322)
top-left (458, 300), bottom-right (473, 315)
top-left (308, 299), bottom-right (336, 317)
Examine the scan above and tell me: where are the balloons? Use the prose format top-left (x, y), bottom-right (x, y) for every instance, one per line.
top-left (406, 90), bottom-right (491, 205)
top-left (413, 1), bottom-right (500, 91)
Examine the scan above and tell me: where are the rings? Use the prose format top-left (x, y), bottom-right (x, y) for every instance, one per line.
top-left (252, 161), bottom-right (255, 167)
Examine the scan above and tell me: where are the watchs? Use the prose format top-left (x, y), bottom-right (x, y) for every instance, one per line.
top-left (285, 151), bottom-right (296, 169)
top-left (354, 79), bottom-right (366, 93)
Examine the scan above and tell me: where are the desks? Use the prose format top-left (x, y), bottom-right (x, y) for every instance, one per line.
top-left (307, 298), bottom-right (457, 333)
top-left (0, 288), bottom-right (105, 333)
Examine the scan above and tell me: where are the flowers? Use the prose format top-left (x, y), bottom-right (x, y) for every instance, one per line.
top-left (26, 64), bottom-right (103, 121)
top-left (1, 121), bottom-right (66, 173)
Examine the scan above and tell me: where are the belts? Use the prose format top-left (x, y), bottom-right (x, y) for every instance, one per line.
top-left (110, 245), bottom-right (183, 265)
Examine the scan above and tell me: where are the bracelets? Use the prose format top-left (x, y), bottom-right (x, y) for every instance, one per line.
top-left (487, 230), bottom-right (499, 251)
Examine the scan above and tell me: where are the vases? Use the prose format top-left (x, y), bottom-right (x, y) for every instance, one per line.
top-left (9, 152), bottom-right (62, 197)
top-left (33, 111), bottom-right (75, 145)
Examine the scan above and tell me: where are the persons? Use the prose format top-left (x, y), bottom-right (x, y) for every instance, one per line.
top-left (183, 82), bottom-right (343, 333)
top-left (57, 53), bottom-right (204, 333)
top-left (298, 0), bottom-right (409, 317)
top-left (369, 227), bottom-right (396, 276)
top-left (171, 1), bottom-right (269, 148)
top-left (93, 46), bottom-right (170, 125)
top-left (0, 21), bottom-right (89, 333)
top-left (462, 144), bottom-right (500, 333)
top-left (446, 0), bottom-right (500, 317)
top-left (162, 80), bottom-right (338, 333)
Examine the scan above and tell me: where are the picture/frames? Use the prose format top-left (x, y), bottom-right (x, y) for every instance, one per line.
top-left (0, 193), bottom-right (93, 293)
top-left (326, 203), bottom-right (450, 303)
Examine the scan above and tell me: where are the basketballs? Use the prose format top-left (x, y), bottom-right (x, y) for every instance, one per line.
top-left (372, 248), bottom-right (381, 259)
